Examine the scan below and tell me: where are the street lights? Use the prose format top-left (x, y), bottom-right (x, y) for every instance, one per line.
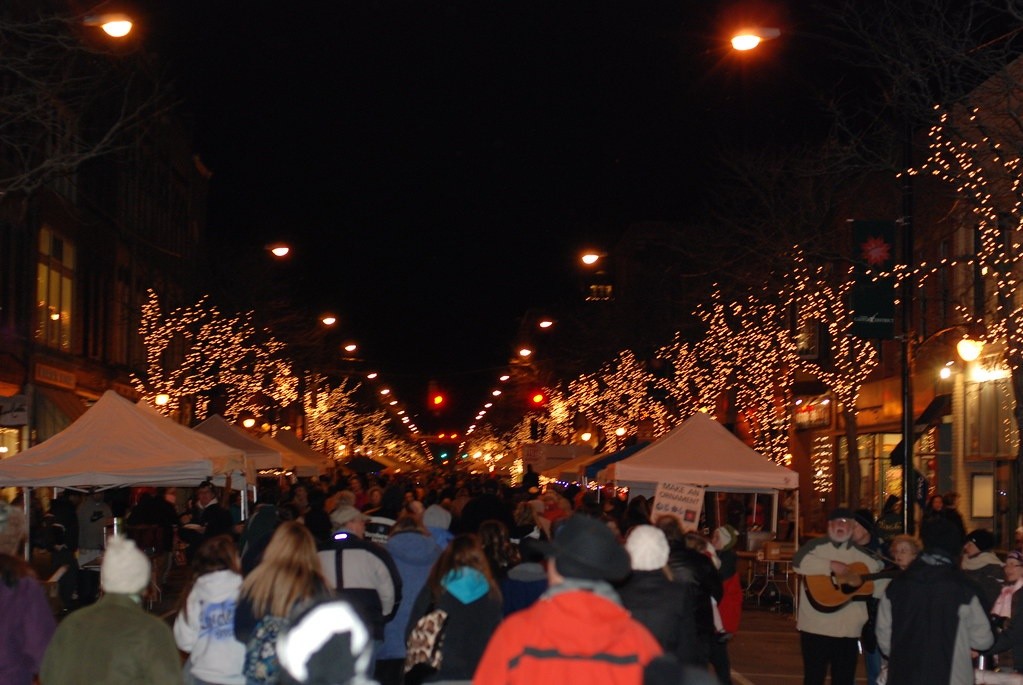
top-left (728, 22), bottom-right (915, 536)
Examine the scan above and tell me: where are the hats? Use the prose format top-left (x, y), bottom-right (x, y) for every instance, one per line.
top-left (828, 508), bottom-right (852, 519)
top-left (714, 525), bottom-right (739, 550)
top-left (329, 506), bottom-right (358, 523)
top-left (100, 534), bottom-right (152, 592)
top-left (531, 514), bottom-right (631, 581)
top-left (625, 525), bottom-right (671, 571)
top-left (1007, 549), bottom-right (1023, 562)
top-left (968, 528), bottom-right (993, 552)
top-left (920, 517), bottom-right (962, 553)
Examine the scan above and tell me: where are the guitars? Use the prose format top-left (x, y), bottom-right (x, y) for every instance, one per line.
top-left (803, 561), bottom-right (905, 613)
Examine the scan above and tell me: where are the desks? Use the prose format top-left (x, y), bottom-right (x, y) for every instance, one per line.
top-left (735, 550), bottom-right (794, 612)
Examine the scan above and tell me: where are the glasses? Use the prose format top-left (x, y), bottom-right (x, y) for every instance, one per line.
top-left (1000, 562), bottom-right (1023, 568)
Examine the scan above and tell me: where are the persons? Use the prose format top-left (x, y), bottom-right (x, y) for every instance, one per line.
top-left (0, 469), bottom-right (403, 684)
top-left (792, 491), bottom-right (1023, 685)
top-left (376, 465), bottom-right (743, 685)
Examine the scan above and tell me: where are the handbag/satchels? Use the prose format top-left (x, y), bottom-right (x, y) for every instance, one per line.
top-left (403, 610), bottom-right (449, 685)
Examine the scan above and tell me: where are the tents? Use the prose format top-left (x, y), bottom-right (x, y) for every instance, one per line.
top-left (0, 390), bottom-right (333, 561)
top-left (544, 411), bottom-right (799, 552)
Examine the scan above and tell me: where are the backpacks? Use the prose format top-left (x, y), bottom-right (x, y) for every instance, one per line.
top-left (243, 615), bottom-right (288, 685)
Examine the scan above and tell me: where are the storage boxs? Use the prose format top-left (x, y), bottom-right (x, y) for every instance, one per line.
top-left (766, 541), bottom-right (795, 560)
top-left (747, 530), bottom-right (773, 551)
